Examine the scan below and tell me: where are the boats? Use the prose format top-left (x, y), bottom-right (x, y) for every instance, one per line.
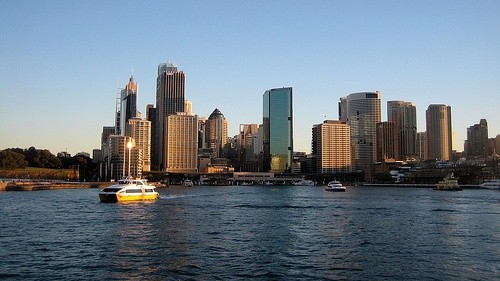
top-left (183, 179), bottom-right (193, 187)
top-left (325, 181), bottom-right (346, 192)
top-left (433, 171), bottom-right (463, 191)
top-left (99, 178), bottom-right (160, 202)
